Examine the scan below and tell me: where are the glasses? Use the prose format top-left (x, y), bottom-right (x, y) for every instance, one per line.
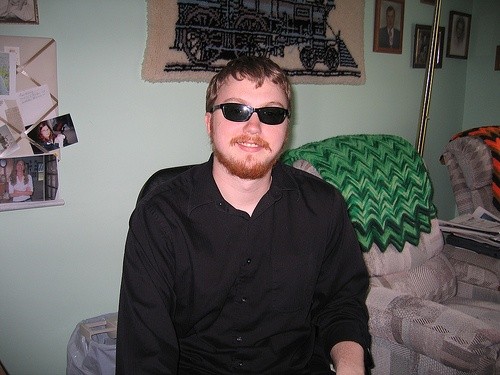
top-left (212, 103), bottom-right (291, 125)
top-left (17, 163), bottom-right (23, 165)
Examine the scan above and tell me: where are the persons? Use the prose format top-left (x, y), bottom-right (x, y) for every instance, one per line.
top-left (453, 16), bottom-right (466, 55)
top-left (379, 5), bottom-right (400, 49)
top-left (115, 55), bottom-right (371, 374)
top-left (8, 160), bottom-right (33, 202)
top-left (38, 124), bottom-right (69, 152)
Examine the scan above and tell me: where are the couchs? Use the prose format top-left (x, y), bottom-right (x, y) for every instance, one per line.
top-left (278, 133), bottom-right (500, 375)
top-left (440, 125), bottom-right (500, 220)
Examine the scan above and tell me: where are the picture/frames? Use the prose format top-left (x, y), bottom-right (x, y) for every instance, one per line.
top-left (446, 10), bottom-right (472, 59)
top-left (413, 24), bottom-right (445, 68)
top-left (373, 0), bottom-right (405, 54)
